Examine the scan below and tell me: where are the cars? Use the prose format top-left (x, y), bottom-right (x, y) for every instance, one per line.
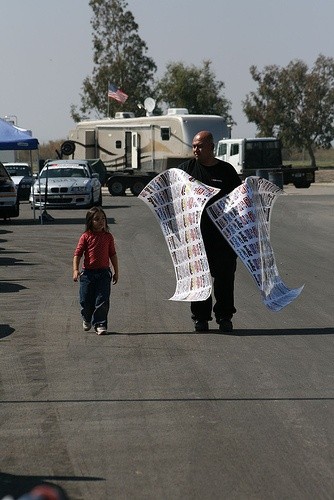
top-left (1, 161), bottom-right (37, 201)
top-left (29, 160), bottom-right (102, 209)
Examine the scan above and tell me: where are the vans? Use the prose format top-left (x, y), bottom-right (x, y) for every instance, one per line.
top-left (0, 159), bottom-right (20, 219)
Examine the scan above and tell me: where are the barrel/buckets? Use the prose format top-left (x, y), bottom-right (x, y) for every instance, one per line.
top-left (268, 172), bottom-right (283, 191)
top-left (256, 169), bottom-right (269, 181)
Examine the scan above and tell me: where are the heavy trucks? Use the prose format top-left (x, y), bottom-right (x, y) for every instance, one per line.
top-left (69, 97), bottom-right (284, 197)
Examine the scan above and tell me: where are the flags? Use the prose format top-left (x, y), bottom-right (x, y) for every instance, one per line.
top-left (108, 83), bottom-right (128, 104)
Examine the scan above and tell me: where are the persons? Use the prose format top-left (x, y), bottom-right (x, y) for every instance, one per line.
top-left (73, 207), bottom-right (119, 334)
top-left (173, 131), bottom-right (243, 331)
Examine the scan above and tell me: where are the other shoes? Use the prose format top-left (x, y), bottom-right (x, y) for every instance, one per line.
top-left (219, 321), bottom-right (233, 331)
top-left (194, 319), bottom-right (209, 331)
top-left (96, 326), bottom-right (108, 335)
top-left (83, 320), bottom-right (92, 331)
